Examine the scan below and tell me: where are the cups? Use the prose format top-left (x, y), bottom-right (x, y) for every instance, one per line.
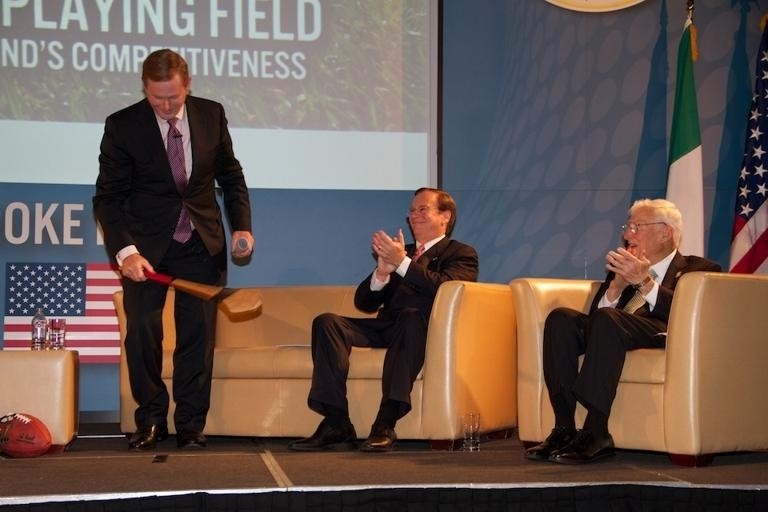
top-left (49, 316), bottom-right (68, 349)
top-left (462, 410), bottom-right (480, 451)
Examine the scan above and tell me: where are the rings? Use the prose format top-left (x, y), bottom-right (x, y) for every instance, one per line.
top-left (378, 245), bottom-right (381, 250)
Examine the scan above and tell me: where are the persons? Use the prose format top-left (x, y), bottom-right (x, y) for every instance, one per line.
top-left (523, 198), bottom-right (722, 466)
top-left (93, 48), bottom-right (255, 450)
top-left (287, 187), bottom-right (478, 452)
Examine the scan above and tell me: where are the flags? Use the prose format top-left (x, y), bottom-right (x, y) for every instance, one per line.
top-left (2, 263), bottom-right (125, 364)
top-left (633, 0), bottom-right (768, 275)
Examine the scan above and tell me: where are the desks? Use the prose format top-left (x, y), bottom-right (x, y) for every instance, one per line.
top-left (0, 349), bottom-right (81, 447)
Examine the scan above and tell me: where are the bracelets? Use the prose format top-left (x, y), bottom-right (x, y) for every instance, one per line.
top-left (632, 277), bottom-right (650, 290)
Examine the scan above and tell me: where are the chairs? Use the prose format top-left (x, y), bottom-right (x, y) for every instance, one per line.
top-left (511, 270), bottom-right (767, 469)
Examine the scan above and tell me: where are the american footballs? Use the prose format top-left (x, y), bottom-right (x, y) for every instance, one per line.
top-left (0, 413), bottom-right (52, 458)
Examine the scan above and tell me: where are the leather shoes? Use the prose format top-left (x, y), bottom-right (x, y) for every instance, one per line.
top-left (177, 432), bottom-right (206, 450)
top-left (528, 431), bottom-right (570, 459)
top-left (128, 421), bottom-right (169, 453)
top-left (288, 419), bottom-right (357, 450)
top-left (360, 424), bottom-right (398, 451)
top-left (548, 433), bottom-right (615, 465)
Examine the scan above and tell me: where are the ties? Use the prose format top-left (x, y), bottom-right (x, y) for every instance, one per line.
top-left (411, 244), bottom-right (425, 263)
top-left (623, 272), bottom-right (658, 314)
top-left (164, 115), bottom-right (192, 245)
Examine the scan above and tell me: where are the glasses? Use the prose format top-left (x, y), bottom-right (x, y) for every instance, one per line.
top-left (620, 222), bottom-right (668, 234)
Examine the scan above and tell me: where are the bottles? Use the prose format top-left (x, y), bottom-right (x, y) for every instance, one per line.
top-left (29, 305), bottom-right (49, 350)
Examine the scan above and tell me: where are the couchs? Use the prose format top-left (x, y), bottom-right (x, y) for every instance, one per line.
top-left (112, 279), bottom-right (517, 452)
